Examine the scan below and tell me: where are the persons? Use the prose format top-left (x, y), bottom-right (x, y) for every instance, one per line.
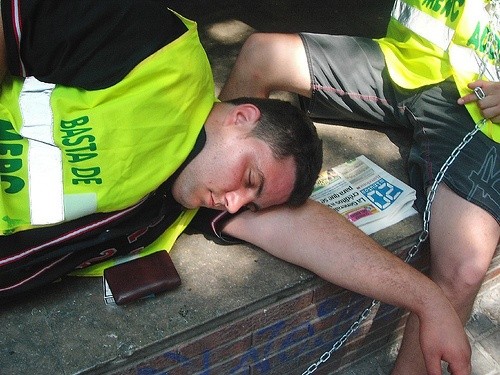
top-left (216, 0), bottom-right (500, 374)
top-left (0, 0), bottom-right (474, 375)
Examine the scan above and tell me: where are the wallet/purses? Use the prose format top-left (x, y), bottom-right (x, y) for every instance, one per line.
top-left (101, 250), bottom-right (181, 304)
